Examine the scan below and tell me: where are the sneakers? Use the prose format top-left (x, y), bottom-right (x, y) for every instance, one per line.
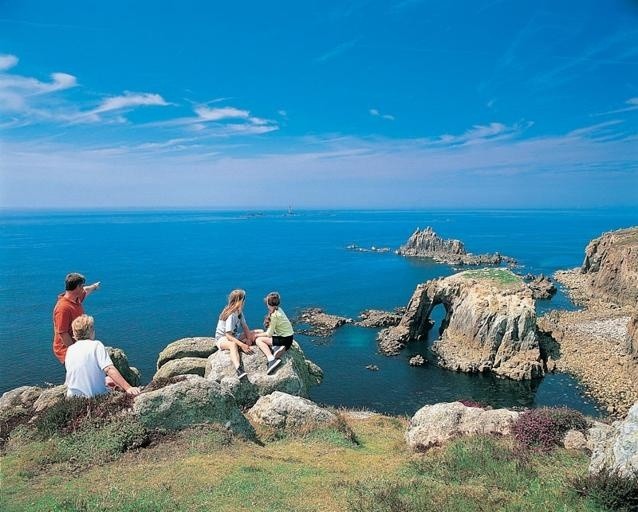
top-left (266, 359), bottom-right (282, 374)
top-left (235, 366), bottom-right (248, 381)
top-left (271, 345), bottom-right (286, 358)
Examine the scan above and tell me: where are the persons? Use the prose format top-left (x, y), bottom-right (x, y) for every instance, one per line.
top-left (213, 288), bottom-right (265, 381)
top-left (52, 271), bottom-right (104, 374)
top-left (249, 291), bottom-right (295, 375)
top-left (65, 313), bottom-right (144, 400)
